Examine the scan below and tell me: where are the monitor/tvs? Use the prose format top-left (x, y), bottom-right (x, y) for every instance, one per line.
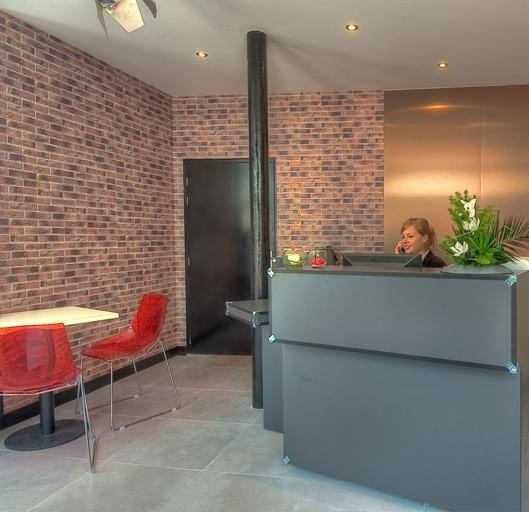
top-left (342, 253), bottom-right (422, 269)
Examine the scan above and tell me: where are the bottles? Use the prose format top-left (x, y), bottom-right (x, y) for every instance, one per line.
top-left (282, 246), bottom-right (328, 268)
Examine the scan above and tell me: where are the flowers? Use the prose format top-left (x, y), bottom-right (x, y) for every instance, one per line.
top-left (437, 189), bottom-right (529, 267)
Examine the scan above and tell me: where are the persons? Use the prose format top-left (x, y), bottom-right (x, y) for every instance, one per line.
top-left (395, 219), bottom-right (447, 268)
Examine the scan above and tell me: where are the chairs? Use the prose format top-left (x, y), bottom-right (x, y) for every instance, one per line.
top-left (0, 291), bottom-right (182, 474)
